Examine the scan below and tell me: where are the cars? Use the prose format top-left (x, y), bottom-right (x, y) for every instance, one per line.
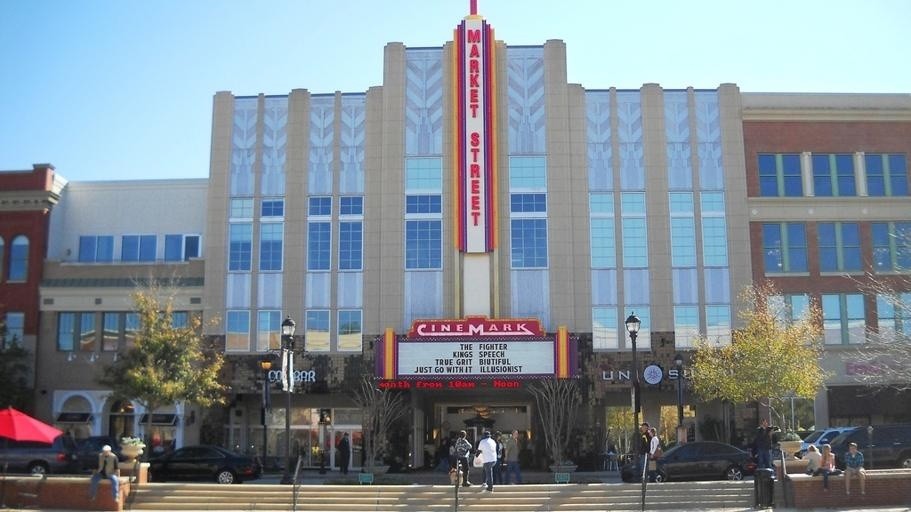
top-left (72, 434), bottom-right (169, 477)
top-left (619, 423), bottom-right (909, 484)
top-left (145, 443), bottom-right (260, 488)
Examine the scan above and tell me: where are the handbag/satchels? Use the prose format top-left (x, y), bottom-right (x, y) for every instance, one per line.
top-left (653, 446), bottom-right (663, 459)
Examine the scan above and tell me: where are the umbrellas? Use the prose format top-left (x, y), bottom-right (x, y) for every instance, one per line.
top-left (0, 405), bottom-right (64, 506)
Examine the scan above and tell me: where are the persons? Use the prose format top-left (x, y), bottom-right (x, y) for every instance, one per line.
top-left (337, 432), bottom-right (350, 474)
top-left (813, 444), bottom-right (835, 492)
top-left (88, 445), bottom-right (120, 502)
top-left (448, 428), bottom-right (522, 493)
top-left (640, 422), bottom-right (652, 481)
top-left (648, 428), bottom-right (659, 482)
top-left (844, 443), bottom-right (866, 495)
top-left (752, 419), bottom-right (780, 468)
top-left (804, 445), bottom-right (822, 476)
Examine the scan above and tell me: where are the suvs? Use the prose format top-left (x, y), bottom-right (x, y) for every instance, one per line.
top-left (1, 433), bottom-right (76, 477)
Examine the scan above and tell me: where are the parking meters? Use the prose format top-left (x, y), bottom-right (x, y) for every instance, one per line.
top-left (864, 425), bottom-right (875, 470)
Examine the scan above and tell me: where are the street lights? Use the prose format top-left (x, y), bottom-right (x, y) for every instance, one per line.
top-left (277, 315), bottom-right (297, 483)
top-left (624, 312), bottom-right (643, 467)
top-left (672, 353), bottom-right (688, 426)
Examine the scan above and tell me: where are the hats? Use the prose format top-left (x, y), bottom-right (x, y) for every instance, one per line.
top-left (484, 430), bottom-right (492, 438)
